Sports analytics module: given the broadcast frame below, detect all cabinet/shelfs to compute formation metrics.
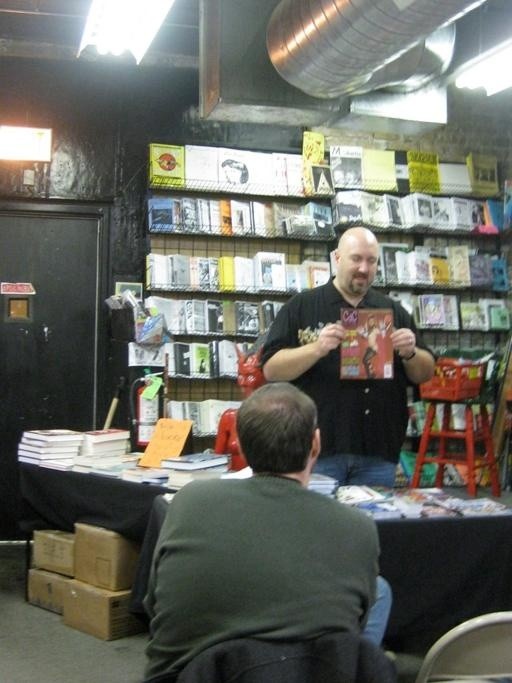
[141,142,512,477]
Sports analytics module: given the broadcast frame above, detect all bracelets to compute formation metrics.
[406,351,416,364]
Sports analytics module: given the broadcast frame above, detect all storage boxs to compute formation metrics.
[61,578,138,640]
[32,528,75,578]
[75,522,138,590]
[26,568,74,615]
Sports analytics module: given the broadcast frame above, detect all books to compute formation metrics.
[148,196,511,237]
[424,334,502,352]
[503,179,511,237]
[402,387,492,433]
[379,239,511,289]
[15,427,254,480]
[150,143,336,196]
[388,292,512,331]
[310,472,511,517]
[167,341,250,380]
[386,148,500,195]
[147,298,285,334]
[165,397,241,433]
[339,307,395,381]
[396,447,503,487]
[328,145,399,192]
[144,250,330,292]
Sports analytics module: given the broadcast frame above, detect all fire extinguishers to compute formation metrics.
[130,368,165,448]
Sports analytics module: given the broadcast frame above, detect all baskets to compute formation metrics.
[419,357,483,402]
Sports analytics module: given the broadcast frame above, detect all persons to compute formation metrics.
[356,315,385,378]
[256,228,434,486]
[143,383,393,682]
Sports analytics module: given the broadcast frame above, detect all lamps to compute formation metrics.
[455,40,512,96]
[75,0,175,65]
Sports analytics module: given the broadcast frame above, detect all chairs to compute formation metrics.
[414,612,512,683]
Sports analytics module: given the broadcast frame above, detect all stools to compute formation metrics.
[409,399,500,498]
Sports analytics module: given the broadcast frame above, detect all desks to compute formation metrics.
[8,458,512,603]
